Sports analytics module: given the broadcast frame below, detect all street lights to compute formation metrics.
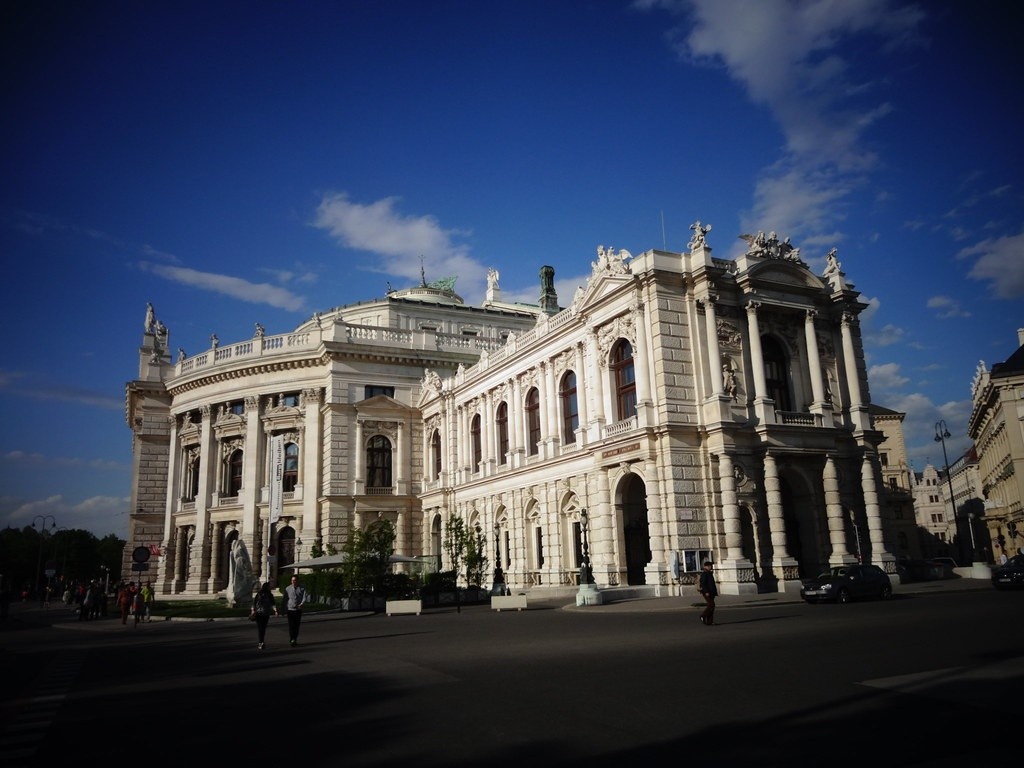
[579,508,596,584]
[32,515,56,592]
[934,420,965,555]
[493,521,507,585]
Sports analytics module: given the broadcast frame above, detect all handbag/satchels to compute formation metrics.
[247,613,256,623]
[115,600,119,608]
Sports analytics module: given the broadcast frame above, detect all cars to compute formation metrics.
[992,554,1024,590]
[800,564,893,605]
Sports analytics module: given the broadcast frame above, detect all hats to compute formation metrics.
[704,562,714,567]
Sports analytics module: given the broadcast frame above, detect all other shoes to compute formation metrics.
[708,621,719,625]
[147,620,152,623]
[122,622,126,624]
[699,615,707,625]
[288,640,297,647]
[141,621,145,623]
[258,642,264,649]
[136,618,139,624]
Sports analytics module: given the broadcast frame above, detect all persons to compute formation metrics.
[697,561,719,625]
[63,580,109,622]
[1000,550,1008,565]
[140,581,155,623]
[282,574,306,647]
[115,582,144,625]
[251,582,278,652]
[41,584,56,608]
